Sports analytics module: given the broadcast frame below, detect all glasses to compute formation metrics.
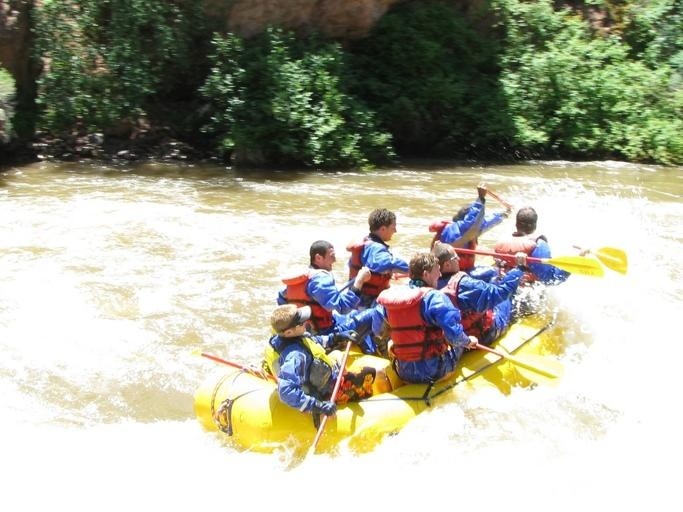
[450,252,458,260]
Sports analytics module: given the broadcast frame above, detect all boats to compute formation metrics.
[193,298,565,463]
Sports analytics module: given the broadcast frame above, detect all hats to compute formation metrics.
[277,305,311,333]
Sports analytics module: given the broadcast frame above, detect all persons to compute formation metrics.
[269,182,573,416]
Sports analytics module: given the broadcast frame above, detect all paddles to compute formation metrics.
[573,245,628,276]
[454,247,603,280]
[477,342,564,386]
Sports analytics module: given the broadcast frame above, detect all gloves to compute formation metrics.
[313,400,337,416]
[333,329,359,342]
